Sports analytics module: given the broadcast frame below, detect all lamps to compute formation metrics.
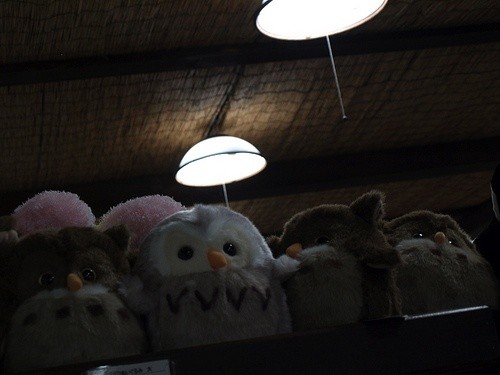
[174,136,269,187]
[254,0,390,42]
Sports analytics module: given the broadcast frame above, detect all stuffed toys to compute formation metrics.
[265,192,408,334]
[98,195,294,350]
[2,190,147,374]
[378,206,498,325]
